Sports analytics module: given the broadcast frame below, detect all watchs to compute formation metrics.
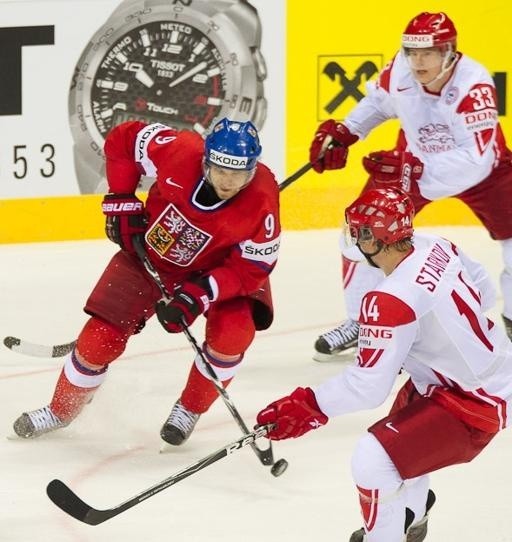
[69,0,268,195]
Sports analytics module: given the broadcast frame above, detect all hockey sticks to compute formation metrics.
[47,423,278,526]
[134,233,273,467]
[4,140,341,358]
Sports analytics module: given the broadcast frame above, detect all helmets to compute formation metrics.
[401,12,457,70]
[202,117,261,187]
[342,187,415,249]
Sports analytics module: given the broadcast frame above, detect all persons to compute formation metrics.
[309,11,512,363]
[6,117,281,454]
[256,185,512,542]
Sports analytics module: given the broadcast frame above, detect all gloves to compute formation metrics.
[253,388,328,441]
[155,277,212,333]
[101,195,150,259]
[309,120,350,173]
[362,152,424,186]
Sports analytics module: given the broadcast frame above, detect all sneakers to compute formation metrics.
[14,404,68,438]
[162,399,202,446]
[315,319,361,356]
[502,314,512,343]
[350,490,436,541]
